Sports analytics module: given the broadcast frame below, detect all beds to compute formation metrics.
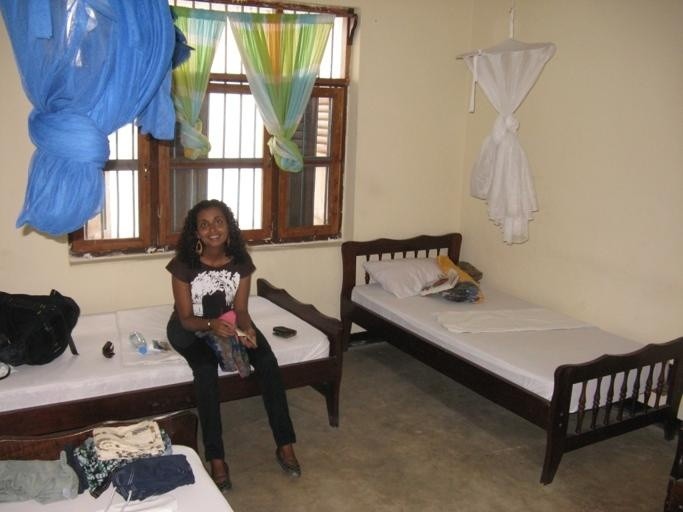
[0,279,344,426]
[0,411,236,512]
[341,233,683,485]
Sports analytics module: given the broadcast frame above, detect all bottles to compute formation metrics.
[128,330,148,355]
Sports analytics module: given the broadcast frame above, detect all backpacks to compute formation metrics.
[0,288,81,367]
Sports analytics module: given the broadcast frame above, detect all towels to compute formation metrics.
[115,303,183,367]
[431,307,598,335]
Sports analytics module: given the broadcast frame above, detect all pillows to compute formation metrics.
[364,258,447,297]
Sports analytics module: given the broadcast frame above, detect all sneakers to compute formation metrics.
[211,459,233,492]
[275,443,301,478]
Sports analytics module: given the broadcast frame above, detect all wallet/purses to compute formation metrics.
[273,325,295,339]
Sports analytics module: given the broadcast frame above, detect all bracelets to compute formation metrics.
[206,319,211,330]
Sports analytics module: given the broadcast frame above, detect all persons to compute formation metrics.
[163,199,303,492]
